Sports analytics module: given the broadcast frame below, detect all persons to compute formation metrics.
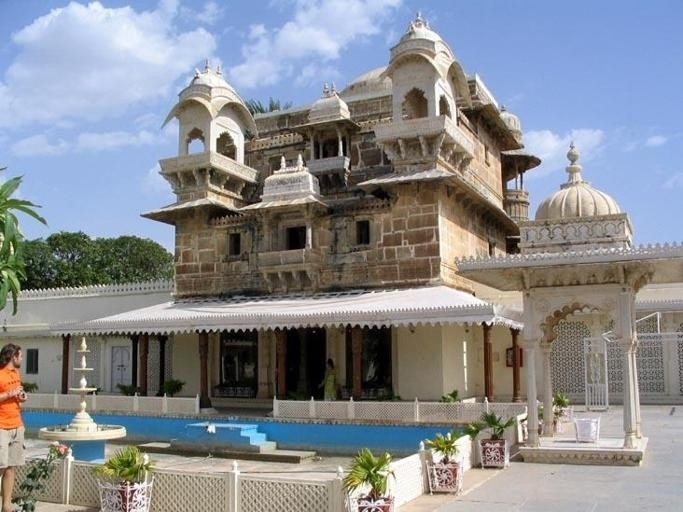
[319,358,338,400]
[0,343,27,512]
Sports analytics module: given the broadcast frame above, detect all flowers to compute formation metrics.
[9,443,71,512]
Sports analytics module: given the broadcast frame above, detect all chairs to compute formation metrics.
[570,414,600,444]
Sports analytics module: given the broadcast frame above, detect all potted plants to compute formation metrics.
[343,442,402,512]
[464,411,517,471]
[534,396,568,438]
[92,440,156,512]
[422,425,467,498]
